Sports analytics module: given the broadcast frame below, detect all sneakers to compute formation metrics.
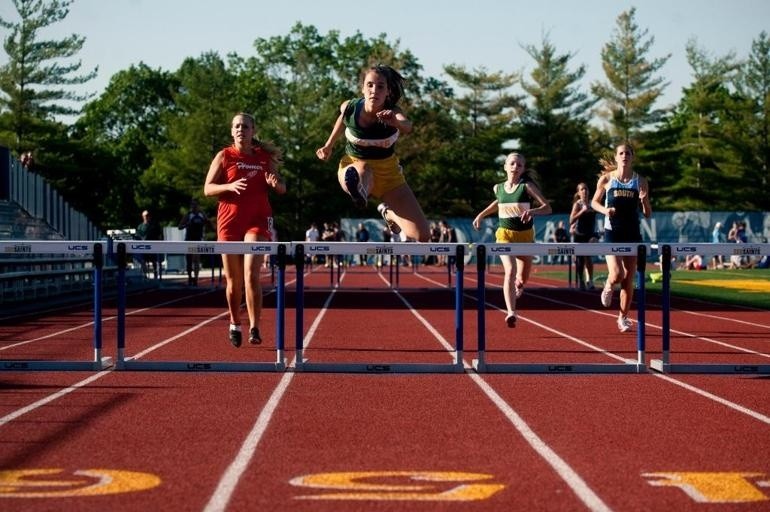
[584,279,634,332]
[378,202,403,235]
[507,282,524,328]
[344,166,370,210]
[228,322,260,349]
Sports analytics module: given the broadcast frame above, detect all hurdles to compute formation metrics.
[112,240,292,371]
[651,242,769,373]
[0,237,114,372]
[473,241,653,373]
[155,252,574,294]
[289,242,470,373]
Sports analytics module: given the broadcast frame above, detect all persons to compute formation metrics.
[179,199,223,285]
[553,221,569,264]
[316,65,431,242]
[137,210,161,281]
[473,152,553,327]
[591,144,650,332]
[305,222,346,267]
[264,254,270,268]
[383,219,456,275]
[570,183,595,290]
[660,222,769,272]
[204,114,286,349]
[356,223,370,266]
